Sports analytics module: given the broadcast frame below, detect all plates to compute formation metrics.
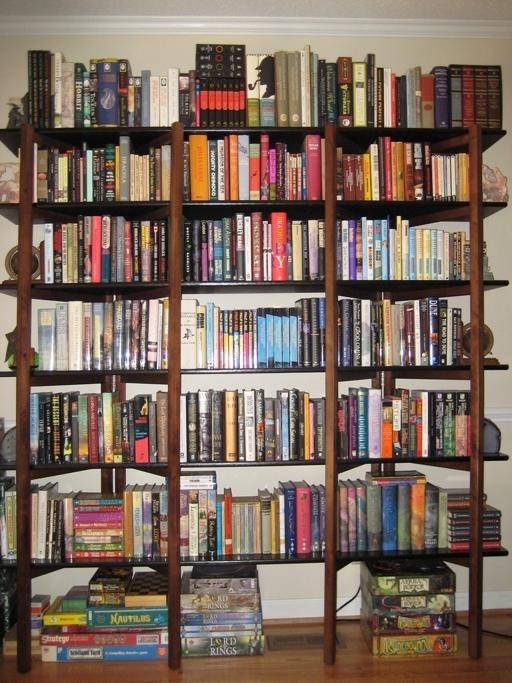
[4,245,41,280]
[463,322,495,358]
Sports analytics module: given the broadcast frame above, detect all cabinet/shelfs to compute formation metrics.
[0,125,509,673]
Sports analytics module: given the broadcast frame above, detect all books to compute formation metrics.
[180,565,265,657]
[3,566,168,662]
[0,42,503,129]
[335,214,502,553]
[180,212,325,556]
[359,559,457,657]
[1,135,471,203]
[31,216,167,558]
[1,476,17,560]
